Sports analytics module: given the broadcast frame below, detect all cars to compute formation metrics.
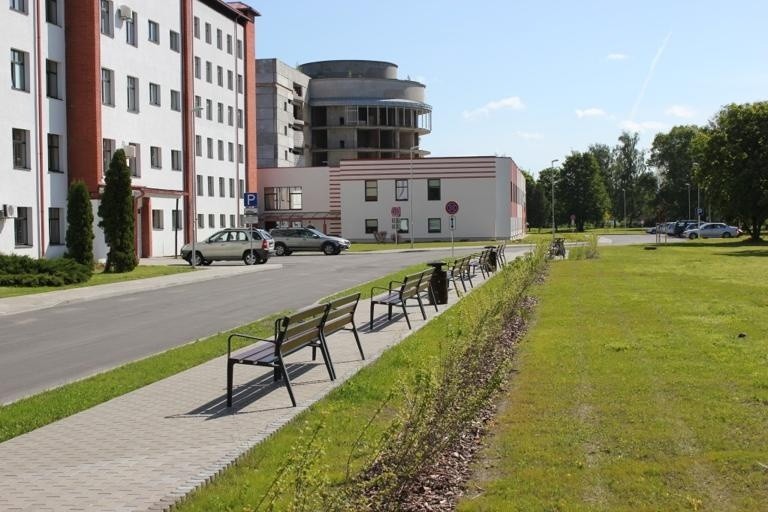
[646,220,743,239]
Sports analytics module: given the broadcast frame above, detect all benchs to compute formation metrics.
[368,244,510,334]
[225,292,365,410]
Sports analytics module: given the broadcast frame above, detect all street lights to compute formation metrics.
[622,189,626,228]
[551,160,558,238]
[685,183,691,219]
[410,145,431,248]
[180,227,351,266]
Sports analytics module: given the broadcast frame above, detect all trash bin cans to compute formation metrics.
[552,238,565,259]
[427,262,448,305]
[483,246,497,272]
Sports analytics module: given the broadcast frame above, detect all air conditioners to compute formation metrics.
[1,203,19,219]
[119,5,133,21]
[124,145,136,159]
